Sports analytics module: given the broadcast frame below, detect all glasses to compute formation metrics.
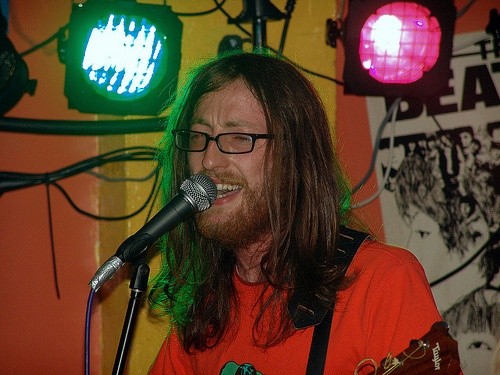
[169,128,287,153]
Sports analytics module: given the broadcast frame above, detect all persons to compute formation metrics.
[147,52,465,375]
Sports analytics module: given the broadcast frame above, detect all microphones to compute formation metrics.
[94,174,218,291]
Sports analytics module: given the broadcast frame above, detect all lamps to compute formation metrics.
[338,0,456,102]
[65,1,183,115]
